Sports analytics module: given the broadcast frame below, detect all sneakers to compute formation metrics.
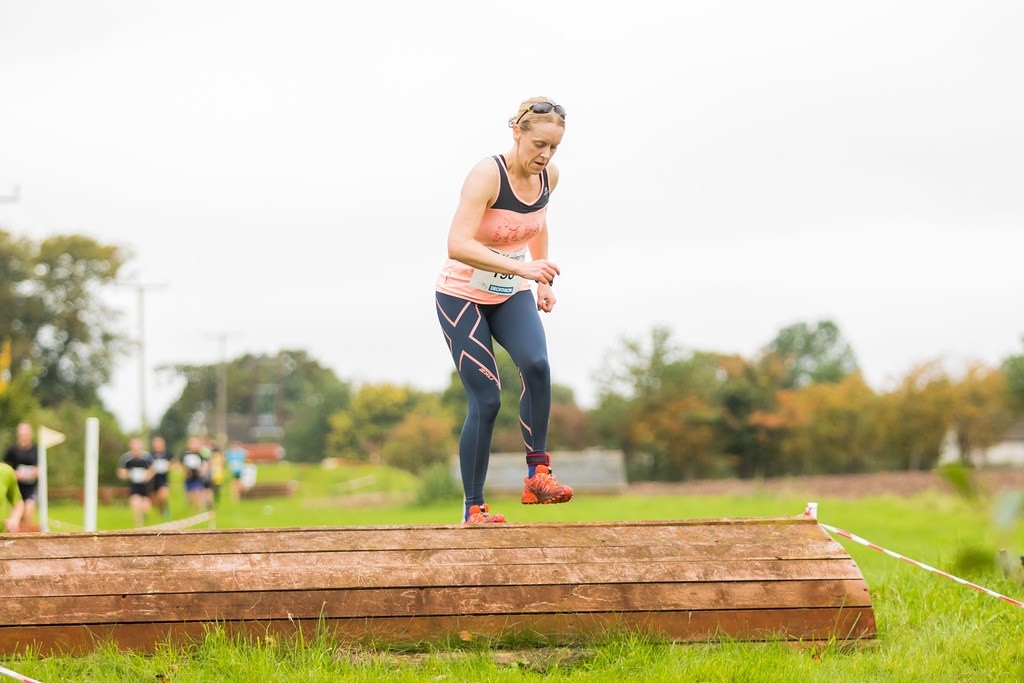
[463,504,506,525]
[521,465,573,504]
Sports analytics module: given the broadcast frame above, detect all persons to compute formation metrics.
[433,95,572,526]
[0,422,254,532]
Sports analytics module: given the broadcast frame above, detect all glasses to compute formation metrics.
[516,102,567,124]
